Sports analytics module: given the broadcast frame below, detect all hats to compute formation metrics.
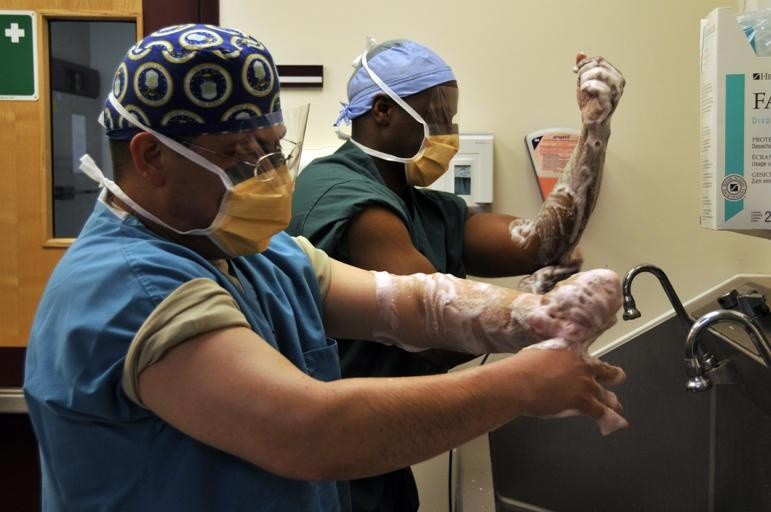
[328,34,458,127]
[96,20,284,140]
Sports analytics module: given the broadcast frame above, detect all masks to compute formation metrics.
[154,133,295,259]
[370,90,459,188]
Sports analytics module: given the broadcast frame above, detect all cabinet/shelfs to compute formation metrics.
[2,2,147,349]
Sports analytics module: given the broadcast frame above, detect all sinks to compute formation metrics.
[487,309,770,512]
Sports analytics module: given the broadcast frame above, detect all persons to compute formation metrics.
[21,21,625,512]
[281,40,625,511]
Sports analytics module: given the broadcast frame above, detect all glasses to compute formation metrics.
[179,140,301,182]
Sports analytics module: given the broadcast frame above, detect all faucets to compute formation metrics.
[686,309,771,394]
[623,263,716,371]
